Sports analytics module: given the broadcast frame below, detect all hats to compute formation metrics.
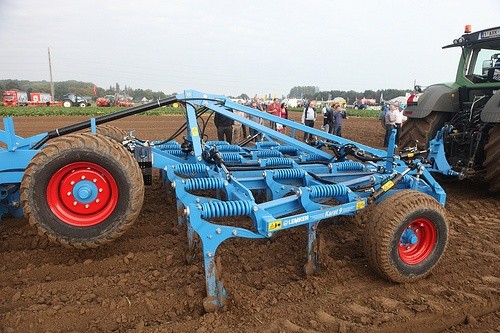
[399,104,405,109]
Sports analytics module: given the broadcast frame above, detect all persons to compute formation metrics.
[238,96,289,145]
[214,102,235,144]
[321,103,404,148]
[301,100,318,142]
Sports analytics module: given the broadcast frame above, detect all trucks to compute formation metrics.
[29,92,51,102]
[96,92,134,107]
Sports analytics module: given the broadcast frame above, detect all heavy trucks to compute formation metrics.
[1,89,28,108]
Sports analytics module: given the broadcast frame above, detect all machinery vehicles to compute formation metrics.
[62,93,91,108]
[397,23,500,196]
